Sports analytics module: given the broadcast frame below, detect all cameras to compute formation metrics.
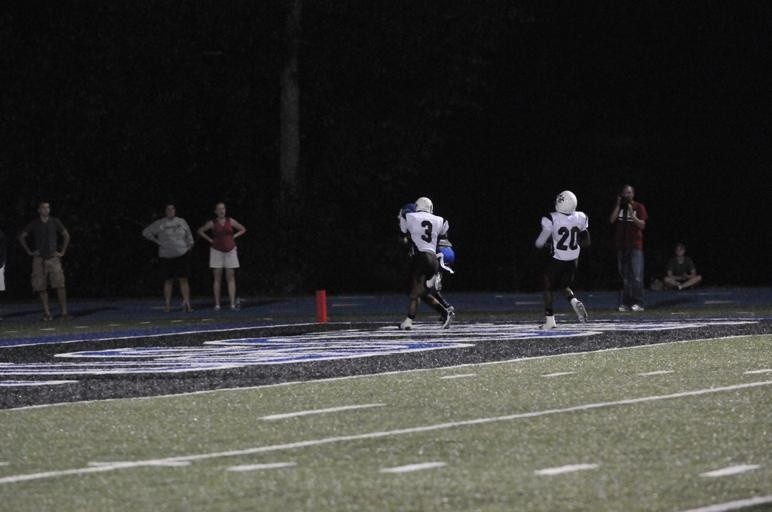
[621,196,629,208]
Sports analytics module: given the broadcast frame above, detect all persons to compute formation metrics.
[0,230,9,321]
[17,200,77,322]
[534,190,592,329]
[661,242,703,291]
[400,201,458,322]
[195,200,248,312]
[141,201,197,314]
[607,184,650,312]
[396,196,454,332]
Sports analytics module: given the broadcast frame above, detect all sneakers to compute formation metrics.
[537,324,557,331]
[398,322,413,331]
[617,304,630,314]
[441,305,455,329]
[59,313,71,323]
[212,307,223,314]
[575,301,589,324]
[230,305,241,312]
[630,304,644,313]
[182,302,195,314]
[162,302,172,315]
[43,313,53,323]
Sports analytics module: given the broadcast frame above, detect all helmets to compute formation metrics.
[554,190,577,215]
[414,196,434,214]
